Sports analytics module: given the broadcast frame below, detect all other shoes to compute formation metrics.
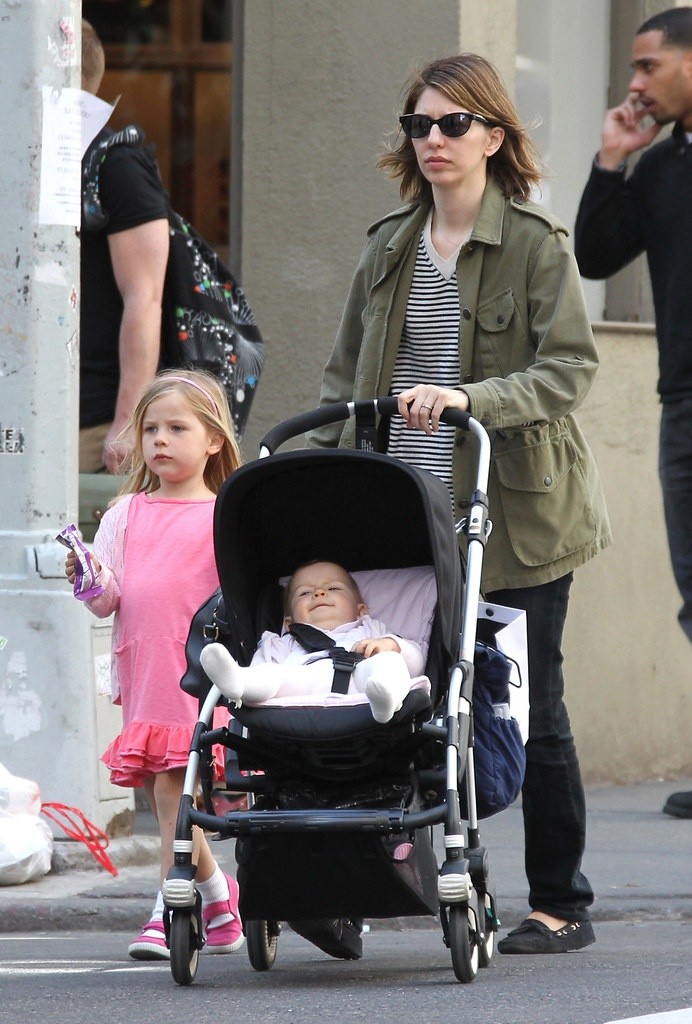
[498,919,597,954]
[288,911,367,960]
[663,790,692,818]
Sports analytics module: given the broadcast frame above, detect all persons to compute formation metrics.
[64,368,245,958]
[197,558,423,724]
[573,5,692,821]
[78,20,177,476]
[287,53,617,960]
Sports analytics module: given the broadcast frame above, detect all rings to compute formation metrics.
[421,404,432,411]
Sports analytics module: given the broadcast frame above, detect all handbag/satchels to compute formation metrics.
[468,642,526,818]
[82,124,264,444]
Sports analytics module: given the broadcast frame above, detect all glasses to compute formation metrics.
[399,112,493,137]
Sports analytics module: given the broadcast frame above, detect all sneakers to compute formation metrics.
[201,873,242,953]
[127,920,171,960]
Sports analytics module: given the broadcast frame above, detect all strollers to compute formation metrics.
[161,397,498,989]
